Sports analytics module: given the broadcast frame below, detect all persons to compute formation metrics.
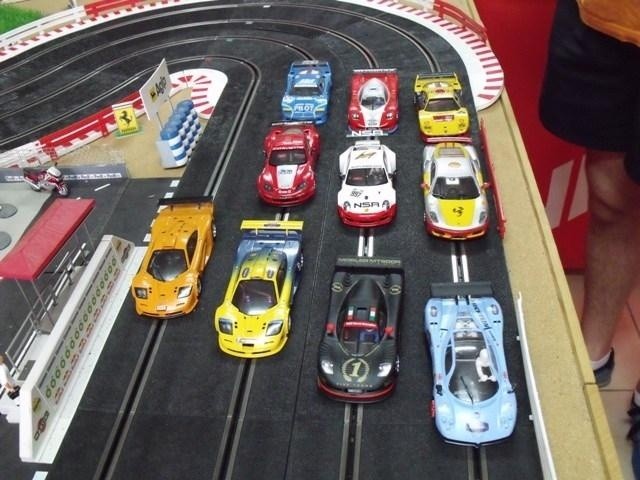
[0,354,20,400]
[538,0,640,480]
